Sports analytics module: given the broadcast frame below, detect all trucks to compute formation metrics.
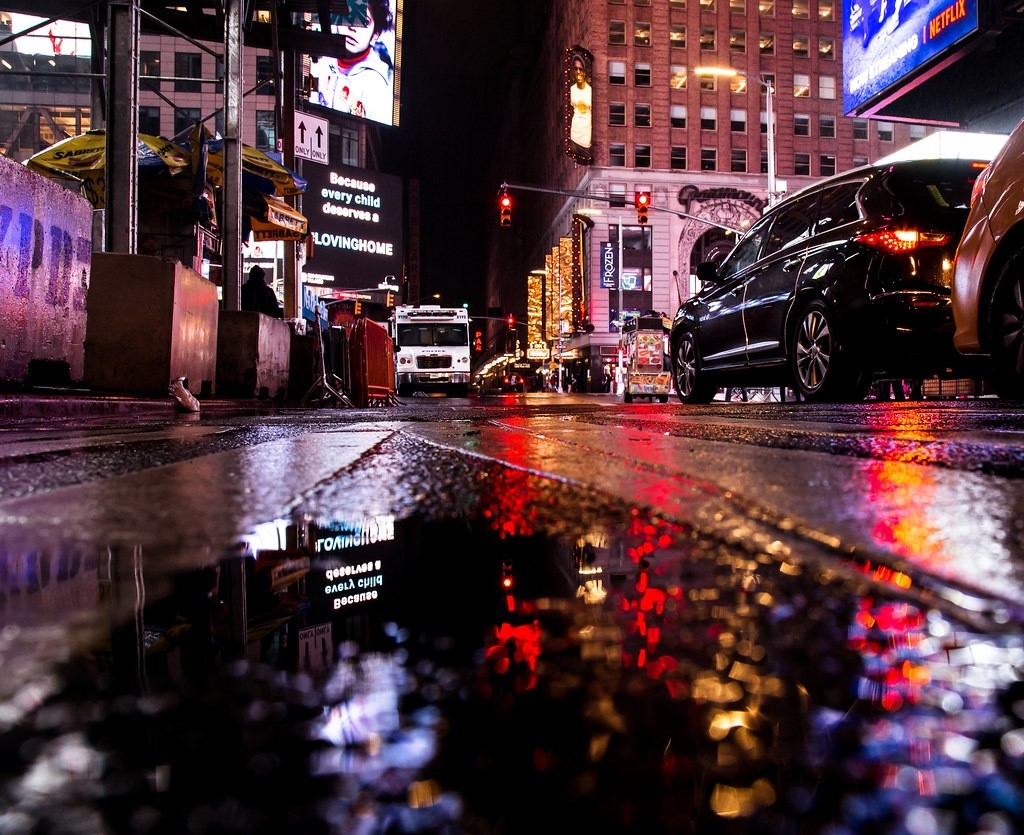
[394,305,472,398]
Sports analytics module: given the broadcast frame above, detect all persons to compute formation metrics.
[570,54,593,156]
[241,265,279,317]
[569,374,576,392]
[604,363,612,380]
[310,0,393,124]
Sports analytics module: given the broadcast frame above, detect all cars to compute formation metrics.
[503,375,524,392]
[949,115,1024,402]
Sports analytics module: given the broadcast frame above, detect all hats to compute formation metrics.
[249,265,265,279]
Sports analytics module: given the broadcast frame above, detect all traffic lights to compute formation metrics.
[463,303,468,308]
[500,196,512,228]
[508,318,513,329]
[637,194,647,223]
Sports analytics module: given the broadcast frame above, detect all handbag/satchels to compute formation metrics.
[22,357,71,394]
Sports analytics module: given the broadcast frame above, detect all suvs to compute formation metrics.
[667,157,991,403]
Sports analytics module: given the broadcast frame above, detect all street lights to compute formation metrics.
[531,270,563,394]
[697,67,774,211]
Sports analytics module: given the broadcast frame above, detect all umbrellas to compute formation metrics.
[22,122,313,243]
